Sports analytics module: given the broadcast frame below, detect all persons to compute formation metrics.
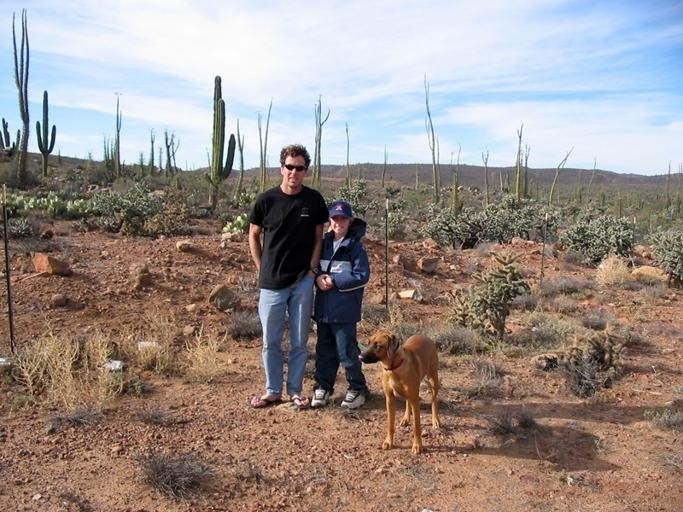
[246,144,329,408]
[309,201,369,411]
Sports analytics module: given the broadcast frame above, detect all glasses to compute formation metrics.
[284,163,307,172]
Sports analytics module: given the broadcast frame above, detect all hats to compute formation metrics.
[330,201,351,218]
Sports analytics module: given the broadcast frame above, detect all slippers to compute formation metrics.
[250,397,311,409]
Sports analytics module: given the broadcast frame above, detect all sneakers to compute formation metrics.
[310,384,370,410]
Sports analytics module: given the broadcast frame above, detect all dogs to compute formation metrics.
[358,330,441,453]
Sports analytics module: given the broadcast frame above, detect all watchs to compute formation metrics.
[310,265,319,274]
